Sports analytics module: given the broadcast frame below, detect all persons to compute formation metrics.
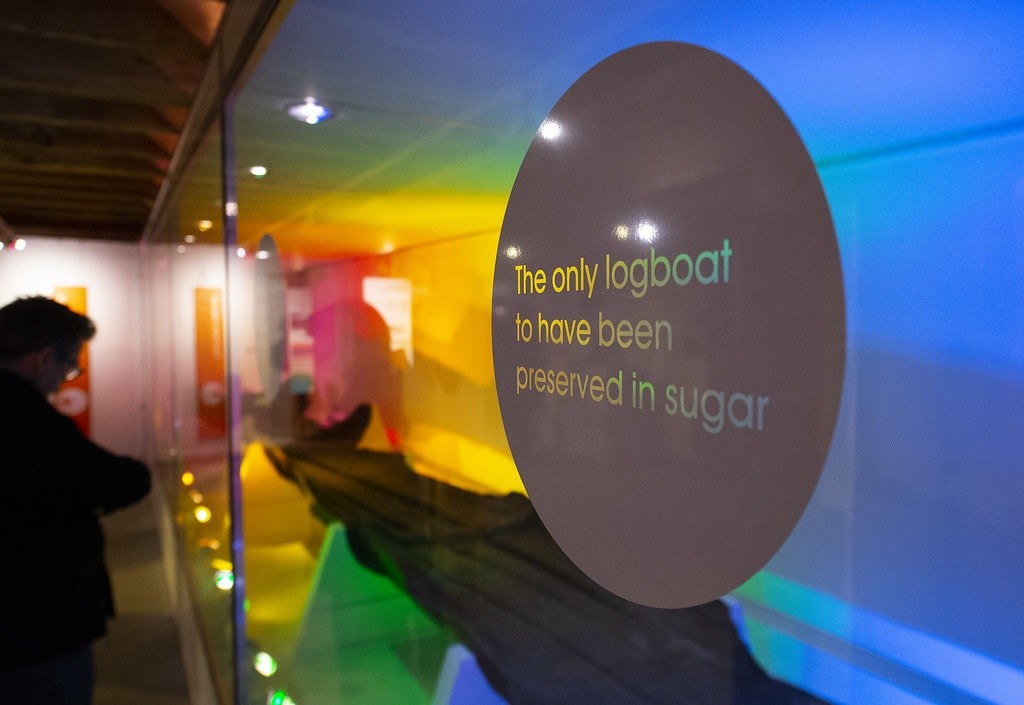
[260,294,433,596]
[0,294,153,705]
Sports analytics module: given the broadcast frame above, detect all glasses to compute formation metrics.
[55,350,81,382]
